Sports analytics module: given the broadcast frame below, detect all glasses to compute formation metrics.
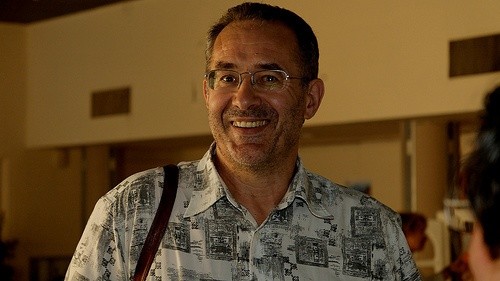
[206,68,309,95]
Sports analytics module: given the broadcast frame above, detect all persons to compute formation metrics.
[398,212,469,281]
[460,84,500,281]
[64,1,422,281]
